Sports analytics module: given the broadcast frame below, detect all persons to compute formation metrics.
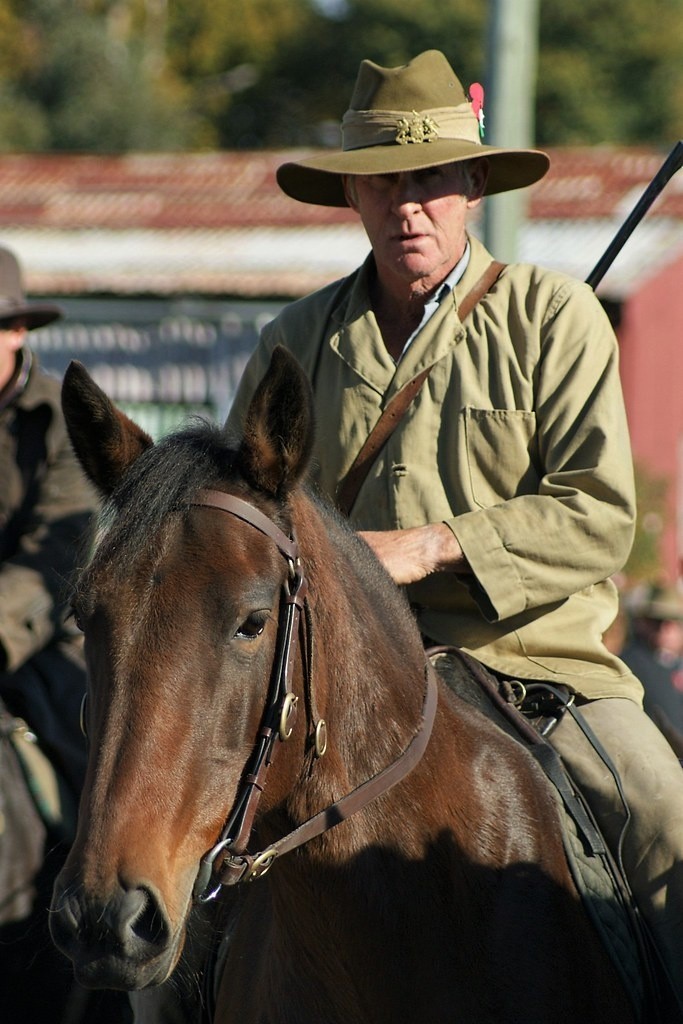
[0,248,98,795]
[618,584,683,767]
[221,49,683,1004]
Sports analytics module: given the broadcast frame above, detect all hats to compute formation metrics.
[0,249,63,330]
[276,48,551,208]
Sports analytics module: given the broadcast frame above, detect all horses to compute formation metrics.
[45,345,681,1023]
